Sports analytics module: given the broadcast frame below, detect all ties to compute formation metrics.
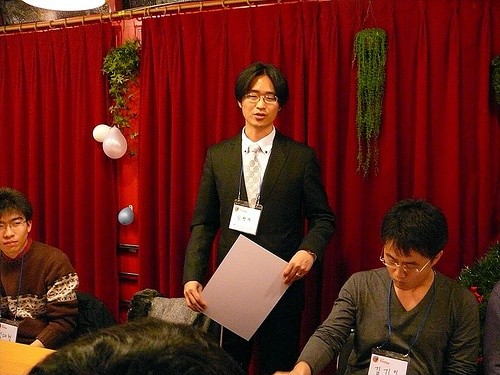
[245,145,261,207]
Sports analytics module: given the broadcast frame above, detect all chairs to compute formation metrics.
[76,292,112,334]
[126,288,217,332]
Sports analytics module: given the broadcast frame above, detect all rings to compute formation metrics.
[296,273,300,276]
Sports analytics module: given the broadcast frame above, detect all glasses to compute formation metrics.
[244,94,278,105]
[0,220,27,233]
[380,248,434,277]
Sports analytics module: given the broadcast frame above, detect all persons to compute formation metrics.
[483,279,500,375]
[182,61,336,375]
[28,317,247,375]
[0,188,81,349]
[276,198,483,375]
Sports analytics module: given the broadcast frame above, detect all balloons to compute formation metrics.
[93,124,111,142]
[103,127,127,159]
[118,207,133,224]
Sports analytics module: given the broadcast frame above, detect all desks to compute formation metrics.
[0,339,57,375]
